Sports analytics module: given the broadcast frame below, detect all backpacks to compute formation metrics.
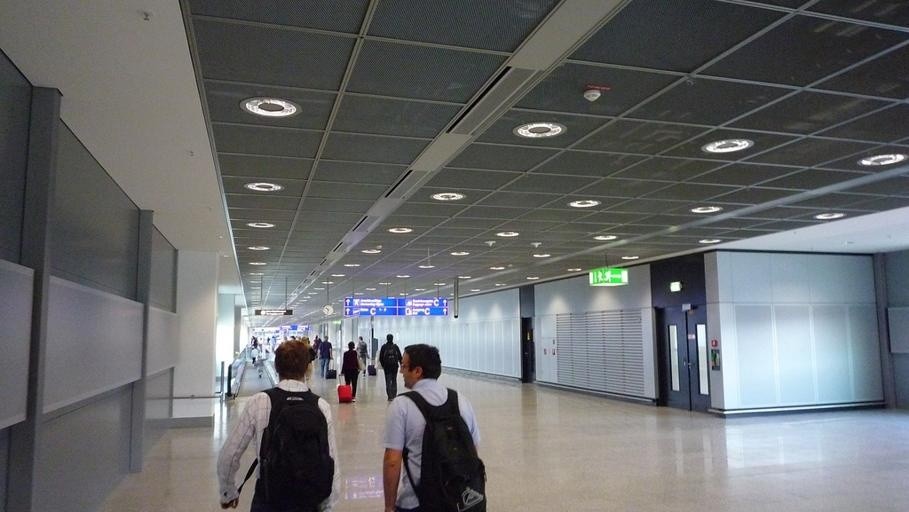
[261,388,335,511]
[397,387,486,512]
[383,344,398,367]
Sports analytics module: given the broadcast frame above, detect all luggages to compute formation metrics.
[368,365,376,375]
[326,369,336,380]
[337,375,352,402]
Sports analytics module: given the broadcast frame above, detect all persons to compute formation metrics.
[250,334,405,405]
[216,339,342,512]
[382,343,486,511]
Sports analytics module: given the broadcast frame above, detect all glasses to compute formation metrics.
[310,348,317,364]
[401,364,406,370]
